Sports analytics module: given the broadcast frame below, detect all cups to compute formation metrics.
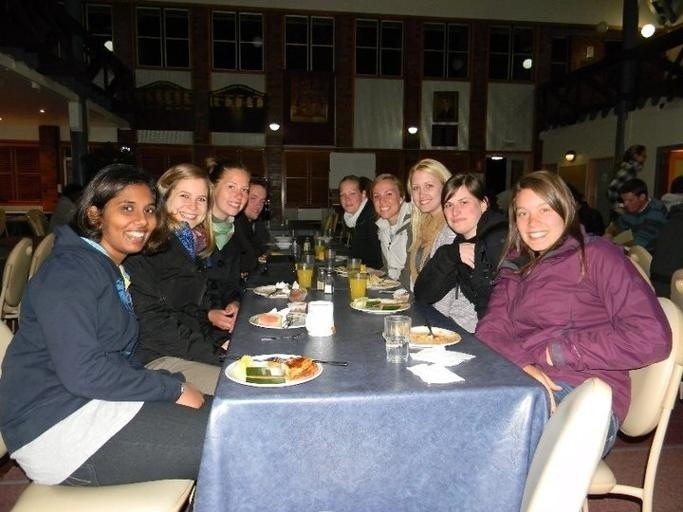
[345,257,361,272]
[313,240,336,269]
[304,299,335,338]
[347,272,367,298]
[295,262,313,288]
[383,315,411,362]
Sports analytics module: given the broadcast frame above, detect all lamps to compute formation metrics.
[431,90,458,122]
[562,149,575,163]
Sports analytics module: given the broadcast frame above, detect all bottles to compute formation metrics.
[316,269,334,294]
[291,235,310,258]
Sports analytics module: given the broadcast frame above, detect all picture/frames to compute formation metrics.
[287,72,331,124]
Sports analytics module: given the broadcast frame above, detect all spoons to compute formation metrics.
[425,319,435,337]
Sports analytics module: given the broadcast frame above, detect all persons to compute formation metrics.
[336,175,383,270]
[0,164,214,488]
[369,173,429,290]
[414,173,516,320]
[205,155,223,182]
[406,158,478,335]
[562,145,683,299]
[474,170,672,459]
[122,163,231,396]
[46,184,82,235]
[206,154,251,335]
[235,180,269,273]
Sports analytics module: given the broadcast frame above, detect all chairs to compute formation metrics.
[514,245,682,512]
[0,205,192,512]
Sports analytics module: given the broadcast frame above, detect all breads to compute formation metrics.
[288,288,306,301]
[257,314,278,326]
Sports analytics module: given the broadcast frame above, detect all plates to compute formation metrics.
[223,353,322,387]
[335,254,347,262]
[273,234,295,250]
[349,298,411,315]
[407,325,464,347]
[248,311,307,330]
[339,266,386,278]
[366,278,400,290]
[252,285,307,299]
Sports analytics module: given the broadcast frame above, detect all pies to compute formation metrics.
[283,358,318,380]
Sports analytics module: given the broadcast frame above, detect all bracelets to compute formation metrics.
[175,384,184,401]
